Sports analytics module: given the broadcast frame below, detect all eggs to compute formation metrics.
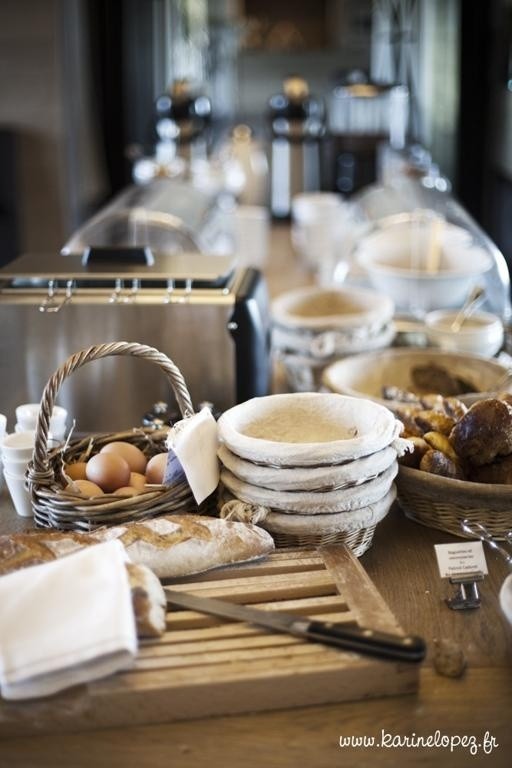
[61,441,170,498]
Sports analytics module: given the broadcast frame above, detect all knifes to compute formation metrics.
[156,587,424,664]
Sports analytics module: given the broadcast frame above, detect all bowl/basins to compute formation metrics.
[265,191,502,394]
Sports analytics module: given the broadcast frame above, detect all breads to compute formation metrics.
[0,510,275,635]
[384,382,511,483]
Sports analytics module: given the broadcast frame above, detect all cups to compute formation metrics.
[1,403,70,519]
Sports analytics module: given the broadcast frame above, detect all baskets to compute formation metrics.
[394,452,512,545]
[208,390,403,557]
[21,340,202,533]
